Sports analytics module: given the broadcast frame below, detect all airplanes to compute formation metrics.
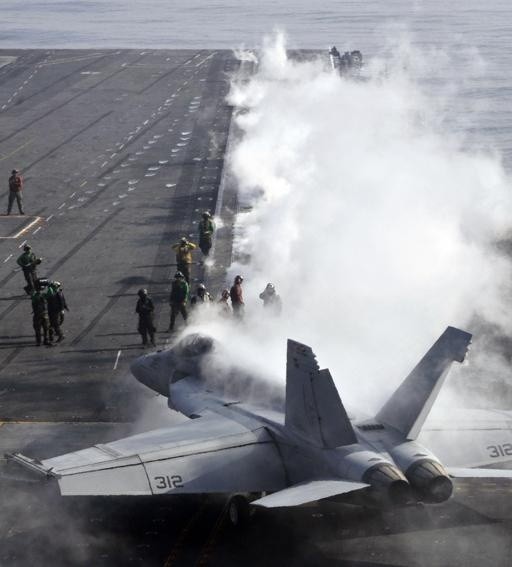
[3,326,512,532]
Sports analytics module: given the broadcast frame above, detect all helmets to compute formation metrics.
[234,275,244,284]
[200,212,211,218]
[10,169,19,174]
[221,290,230,298]
[266,283,275,290]
[23,244,32,250]
[137,288,148,296]
[174,271,185,277]
[179,237,187,243]
[197,284,206,290]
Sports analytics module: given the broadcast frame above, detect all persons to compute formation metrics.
[16,244,41,295]
[171,237,197,288]
[136,288,157,346]
[197,211,214,256]
[32,277,70,347]
[6,170,25,215]
[166,271,282,333]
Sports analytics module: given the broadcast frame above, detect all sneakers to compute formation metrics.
[166,328,176,334]
[141,338,157,348]
[24,286,33,295]
[7,208,25,216]
[37,335,65,346]
